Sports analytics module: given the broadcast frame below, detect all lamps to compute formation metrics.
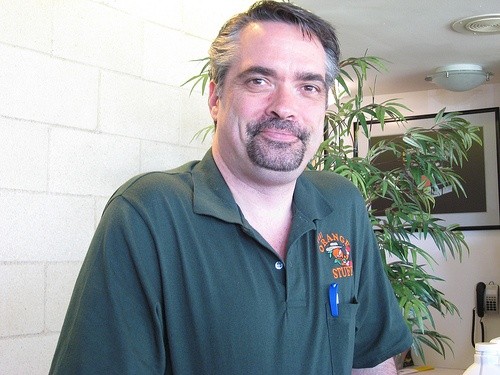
[426,65,495,92]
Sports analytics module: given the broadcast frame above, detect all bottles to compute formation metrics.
[474,343,500,375]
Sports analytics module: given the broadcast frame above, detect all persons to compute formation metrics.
[48,0,414,375]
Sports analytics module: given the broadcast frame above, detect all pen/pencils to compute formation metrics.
[329,283,340,317]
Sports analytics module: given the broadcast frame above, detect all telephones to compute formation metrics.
[476,281,499,318]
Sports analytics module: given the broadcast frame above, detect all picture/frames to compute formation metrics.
[354,106,500,234]
